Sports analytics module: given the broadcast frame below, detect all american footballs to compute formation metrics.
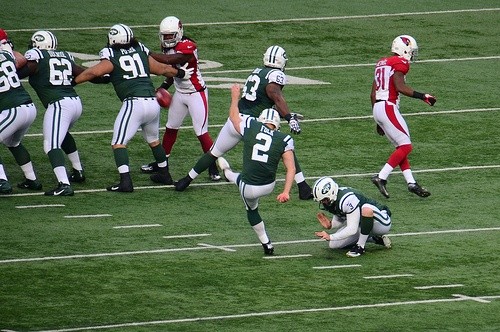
[156,88,172,108]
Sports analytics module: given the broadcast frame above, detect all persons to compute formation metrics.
[174,46,313,199]
[139,17,221,181]
[371,35,437,198]
[310,177,392,258]
[0,29,42,193]
[71,23,194,191]
[218,82,296,255]
[15,31,111,196]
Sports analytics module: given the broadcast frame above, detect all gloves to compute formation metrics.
[142,43,152,55]
[413,91,436,106]
[377,124,385,136]
[284,113,300,135]
[176,62,194,79]
[290,112,304,119]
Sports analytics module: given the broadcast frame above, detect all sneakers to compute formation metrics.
[107,184,133,192]
[346,243,366,257]
[17,178,42,190]
[379,235,391,248]
[371,174,390,198]
[140,157,169,174]
[408,182,434,196]
[175,179,186,191]
[265,244,274,255]
[0,179,13,194]
[44,181,75,196]
[151,172,172,184]
[218,157,231,182]
[68,167,85,182]
[210,174,221,181]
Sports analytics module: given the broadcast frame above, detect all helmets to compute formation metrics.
[31,30,58,50]
[263,45,288,71]
[0,28,8,43]
[392,35,418,63]
[159,15,183,47]
[313,176,339,203]
[107,23,134,47]
